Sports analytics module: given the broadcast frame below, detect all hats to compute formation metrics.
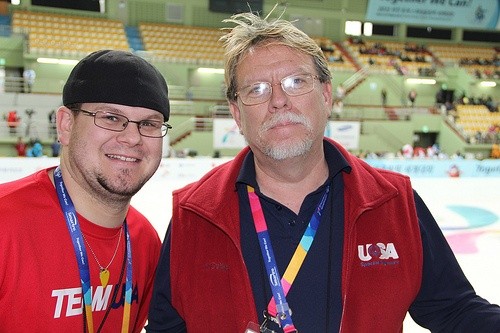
[62,50,170,122]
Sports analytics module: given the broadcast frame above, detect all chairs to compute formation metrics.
[311,36,500,73]
[459,104,500,139]
[11,9,134,60]
[141,19,229,60]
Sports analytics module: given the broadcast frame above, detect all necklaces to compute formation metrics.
[81,227,122,288]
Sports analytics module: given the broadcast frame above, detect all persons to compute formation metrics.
[319,33,500,161]
[22,65,37,92]
[0,50,173,333]
[6,106,61,157]
[145,12,500,333]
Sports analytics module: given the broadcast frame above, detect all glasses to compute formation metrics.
[72,109,172,137]
[233,73,319,107]
